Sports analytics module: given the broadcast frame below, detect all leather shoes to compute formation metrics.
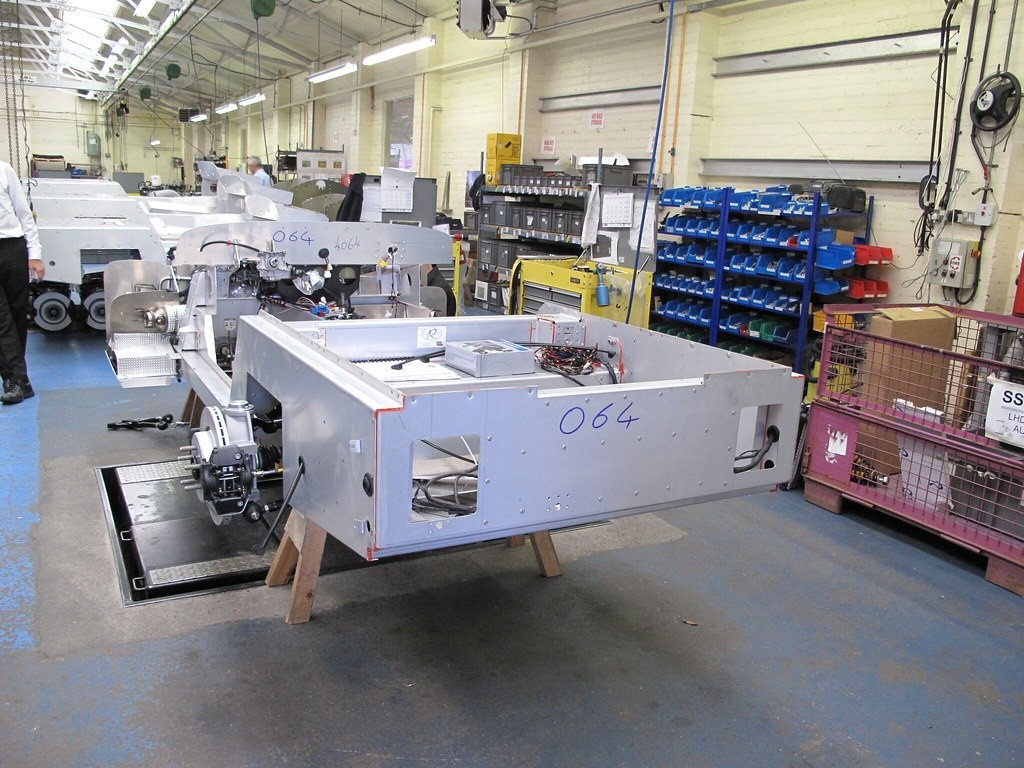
[0,379,34,403]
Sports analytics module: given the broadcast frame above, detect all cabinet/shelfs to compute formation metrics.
[276,143,345,184]
[476,165,635,316]
[713,186,874,373]
[647,200,747,345]
[799,302,1024,598]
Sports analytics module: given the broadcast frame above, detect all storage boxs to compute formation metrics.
[648,185,894,365]
[475,272,511,307]
[482,200,583,237]
[484,132,584,188]
[856,305,958,475]
[948,427,1024,541]
[479,237,551,269]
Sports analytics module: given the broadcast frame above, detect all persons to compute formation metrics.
[0,160,45,403]
[248,156,273,187]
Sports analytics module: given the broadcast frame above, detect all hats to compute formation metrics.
[248,156,260,165]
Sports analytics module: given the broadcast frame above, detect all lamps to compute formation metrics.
[86,37,129,98]
[133,0,157,17]
[214,103,239,114]
[362,34,437,65]
[190,114,208,123]
[307,63,358,83]
[238,94,267,106]
